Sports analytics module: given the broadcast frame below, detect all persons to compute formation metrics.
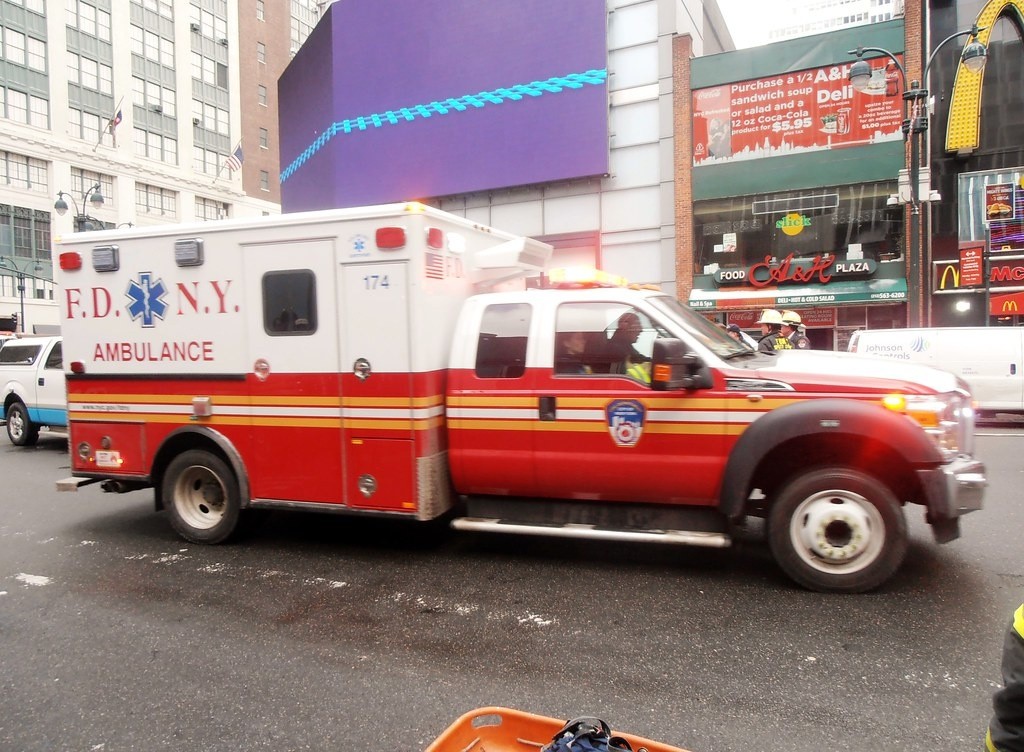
[756,310,795,351]
[607,312,652,374]
[781,311,811,350]
[726,324,754,351]
[555,332,603,374]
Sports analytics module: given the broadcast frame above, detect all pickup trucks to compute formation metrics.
[846,321,1024,424]
[0,334,64,448]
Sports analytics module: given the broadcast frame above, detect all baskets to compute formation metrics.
[421,704,698,752]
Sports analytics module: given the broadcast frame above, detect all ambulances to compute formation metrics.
[58,204,988,595]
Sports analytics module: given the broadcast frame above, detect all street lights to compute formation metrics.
[0,254,49,332]
[55,184,105,232]
[842,25,987,324]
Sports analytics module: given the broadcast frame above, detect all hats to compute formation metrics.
[725,323,741,334]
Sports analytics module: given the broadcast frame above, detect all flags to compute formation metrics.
[113,110,122,128]
[227,146,244,173]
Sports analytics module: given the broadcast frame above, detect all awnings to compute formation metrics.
[689,278,908,311]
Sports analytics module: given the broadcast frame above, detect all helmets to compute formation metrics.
[755,309,789,327]
[780,310,807,329]
